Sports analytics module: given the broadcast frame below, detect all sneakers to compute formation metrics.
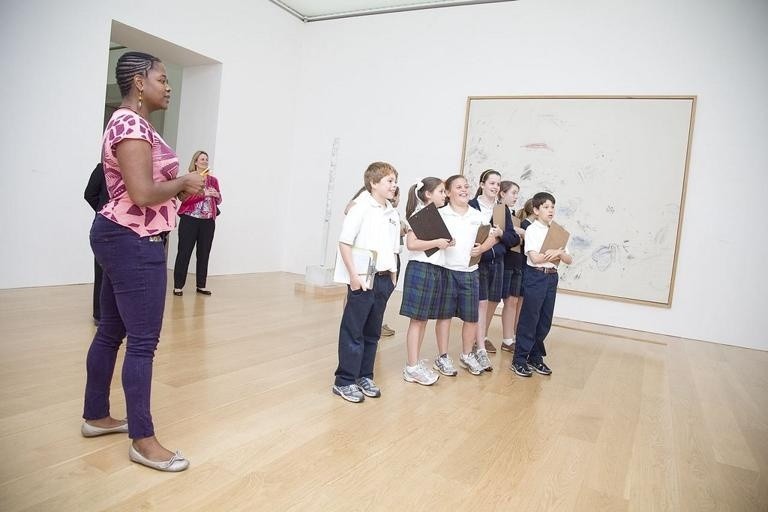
[172,287,184,297]
[484,336,497,354]
[526,359,553,375]
[509,360,532,377]
[355,375,382,398]
[500,340,516,354]
[330,381,366,404]
[126,442,192,474]
[381,324,396,337]
[401,358,440,387]
[474,347,494,372]
[194,288,213,296]
[432,351,459,377]
[458,350,485,375]
[79,415,131,439]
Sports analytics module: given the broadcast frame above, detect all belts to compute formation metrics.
[533,266,558,274]
[377,270,392,277]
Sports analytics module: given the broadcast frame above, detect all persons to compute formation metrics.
[81,51,206,472]
[173,151,222,296]
[84,162,110,327]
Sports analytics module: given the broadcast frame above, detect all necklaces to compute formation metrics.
[119,105,145,118]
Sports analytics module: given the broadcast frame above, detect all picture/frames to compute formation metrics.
[456,94,699,309]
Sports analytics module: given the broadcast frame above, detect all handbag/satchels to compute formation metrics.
[209,175,222,217]
